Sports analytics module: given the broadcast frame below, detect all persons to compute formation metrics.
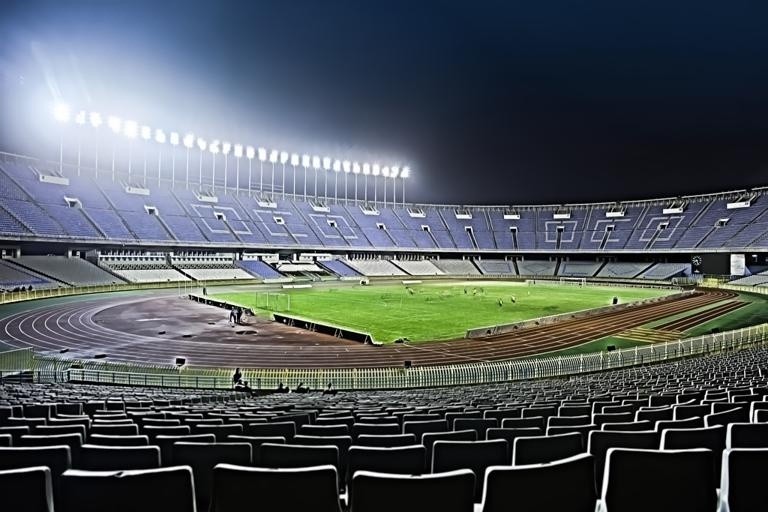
[511,294,516,305]
[463,285,467,295]
[229,307,242,324]
[472,288,477,297]
[498,297,504,308]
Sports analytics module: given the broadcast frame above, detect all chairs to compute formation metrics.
[1,340,767,511]
